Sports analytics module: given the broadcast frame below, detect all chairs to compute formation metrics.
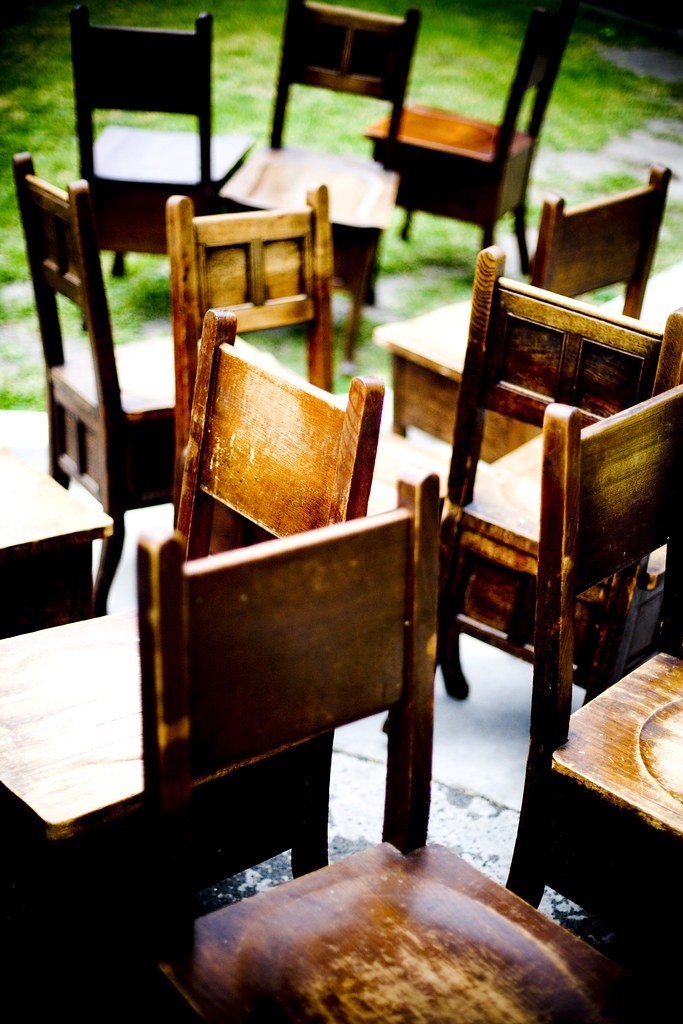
[0,0,683,1024]
